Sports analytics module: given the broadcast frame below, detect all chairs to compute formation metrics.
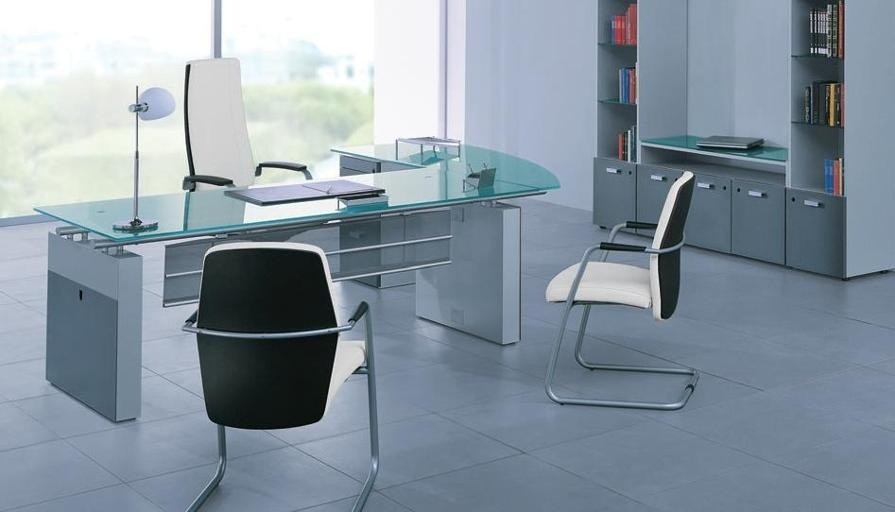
[182,58,312,193]
[544,171,700,410]
[180,241,380,511]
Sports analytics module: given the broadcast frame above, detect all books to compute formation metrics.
[803,1,844,197]
[608,2,637,164]
[224,177,387,207]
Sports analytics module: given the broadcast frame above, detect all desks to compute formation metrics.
[34,137,561,424]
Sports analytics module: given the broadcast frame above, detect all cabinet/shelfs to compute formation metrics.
[592,1,894,282]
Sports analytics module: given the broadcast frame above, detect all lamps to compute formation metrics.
[114,85,176,231]
